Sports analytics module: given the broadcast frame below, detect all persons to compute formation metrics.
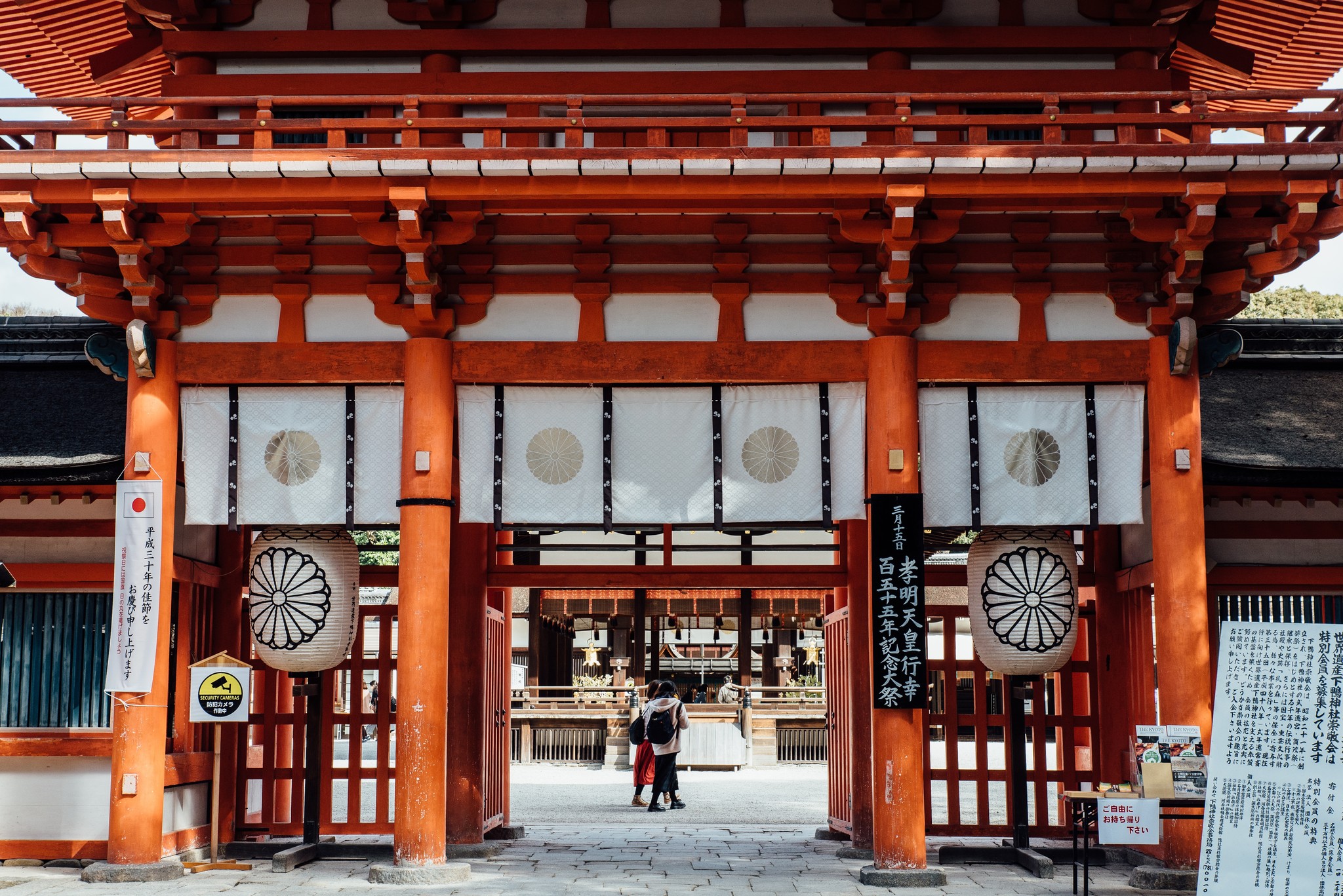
[362,679,371,742]
[694,686,702,703]
[368,680,378,740]
[697,683,709,703]
[631,678,681,808]
[717,675,739,704]
[647,681,690,813]
[683,684,696,703]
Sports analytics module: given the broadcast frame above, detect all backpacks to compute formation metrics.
[629,706,647,745]
[646,702,676,744]
[693,696,702,703]
[723,685,738,704]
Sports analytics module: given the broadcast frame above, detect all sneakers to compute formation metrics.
[632,795,651,807]
[662,792,680,805]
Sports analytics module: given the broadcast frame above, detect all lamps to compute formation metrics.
[581,613,602,666]
[803,617,822,665]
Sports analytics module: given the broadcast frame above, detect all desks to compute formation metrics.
[1057,794,1206,896]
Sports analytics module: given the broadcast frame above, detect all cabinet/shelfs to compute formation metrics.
[1129,735,1207,798]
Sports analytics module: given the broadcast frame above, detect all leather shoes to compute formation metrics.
[648,803,666,812]
[670,799,686,809]
